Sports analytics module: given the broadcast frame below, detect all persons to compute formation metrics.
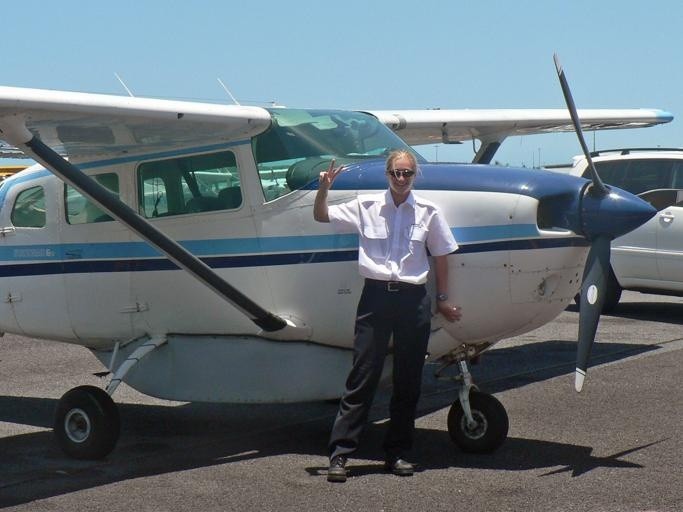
[312,150,463,482]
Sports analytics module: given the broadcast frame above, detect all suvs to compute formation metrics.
[564,142,683,312]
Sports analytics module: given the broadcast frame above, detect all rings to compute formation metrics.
[453,306,456,309]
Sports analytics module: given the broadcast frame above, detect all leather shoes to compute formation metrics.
[328,457,346,482]
[385,455,414,475]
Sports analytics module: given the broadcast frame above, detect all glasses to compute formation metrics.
[387,169,416,178]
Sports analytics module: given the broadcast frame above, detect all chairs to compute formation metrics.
[186,186,241,213]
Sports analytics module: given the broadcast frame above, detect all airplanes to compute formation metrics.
[0,52,678,465]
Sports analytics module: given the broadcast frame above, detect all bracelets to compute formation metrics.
[436,292,448,301]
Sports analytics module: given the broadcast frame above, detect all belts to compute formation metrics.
[365,278,418,292]
